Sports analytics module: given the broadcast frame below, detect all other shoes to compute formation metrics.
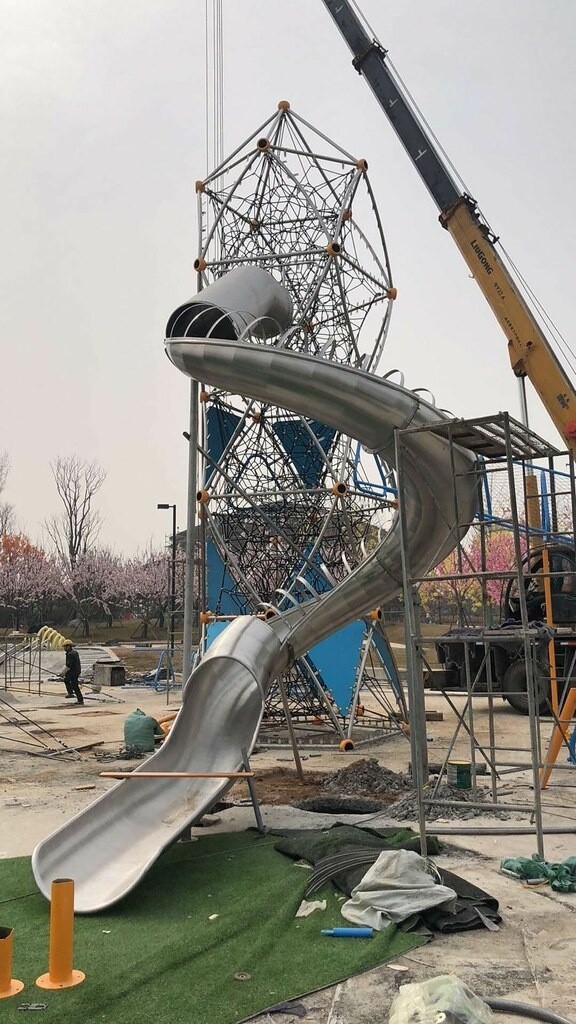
[74,699,84,704]
[65,692,74,698]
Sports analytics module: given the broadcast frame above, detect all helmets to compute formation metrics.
[63,640,73,647]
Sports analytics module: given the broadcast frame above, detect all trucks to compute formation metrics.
[422,539,576,717]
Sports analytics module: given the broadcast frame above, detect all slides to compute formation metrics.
[27,264,484,920]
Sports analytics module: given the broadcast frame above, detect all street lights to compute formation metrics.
[158,503,177,658]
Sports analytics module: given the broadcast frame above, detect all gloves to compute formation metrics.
[59,666,70,677]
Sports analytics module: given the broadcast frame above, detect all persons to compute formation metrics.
[57,639,84,705]
